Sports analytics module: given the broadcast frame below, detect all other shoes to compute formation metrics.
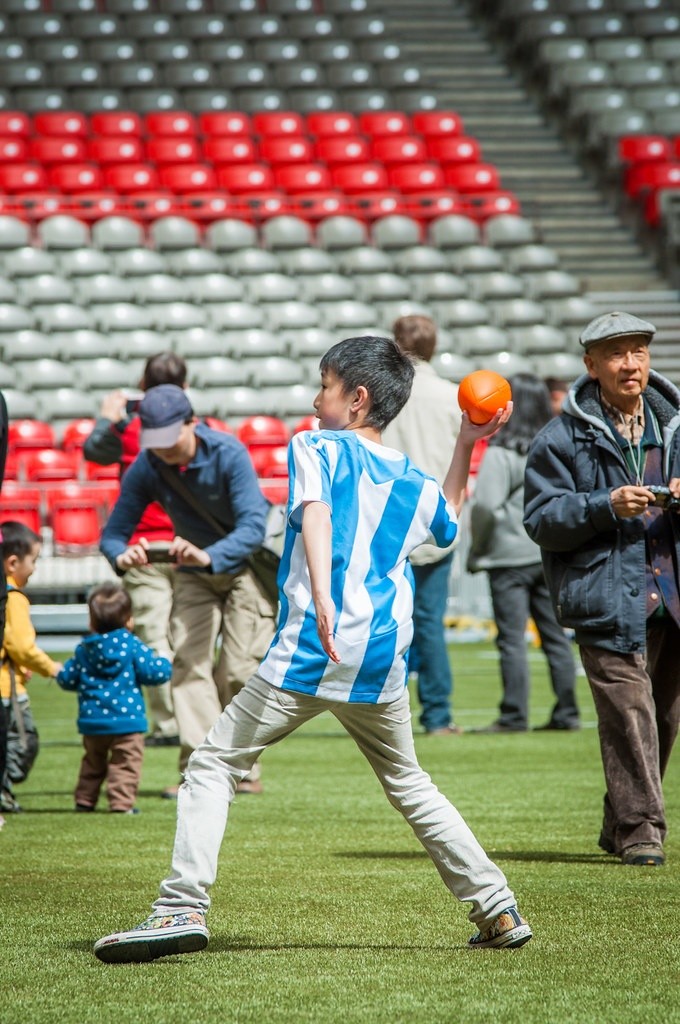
[472,722,527,733]
[533,723,577,731]
[162,780,184,799]
[429,726,461,736]
[75,802,94,812]
[113,808,140,814]
[236,779,262,793]
[598,829,616,854]
[622,843,666,865]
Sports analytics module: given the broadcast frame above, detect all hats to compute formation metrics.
[139,385,193,450]
[579,311,656,350]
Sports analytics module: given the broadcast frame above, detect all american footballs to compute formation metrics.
[459,370,513,426]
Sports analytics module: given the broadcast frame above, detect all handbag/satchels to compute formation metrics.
[250,504,287,602]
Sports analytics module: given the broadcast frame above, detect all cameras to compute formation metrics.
[122,393,144,417]
[646,485,680,512]
[144,542,177,563]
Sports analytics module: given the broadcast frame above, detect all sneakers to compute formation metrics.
[468,909,533,949]
[94,912,209,964]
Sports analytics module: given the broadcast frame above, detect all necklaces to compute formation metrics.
[617,411,643,486]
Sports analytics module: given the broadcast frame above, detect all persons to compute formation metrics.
[55,588,172,814]
[94,337,532,961]
[0,314,680,863]
[467,374,582,733]
